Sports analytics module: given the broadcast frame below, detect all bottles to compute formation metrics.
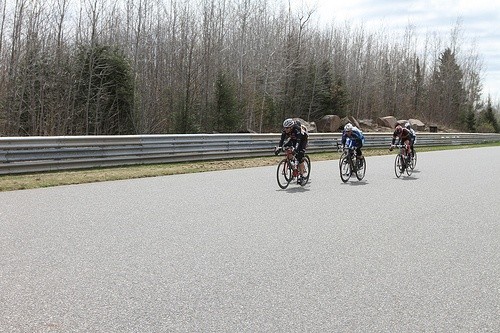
[351,156,356,165]
[290,160,295,166]
[295,160,298,169]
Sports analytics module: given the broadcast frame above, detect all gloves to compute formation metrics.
[275,150,280,155]
[292,149,299,156]
[389,145,394,151]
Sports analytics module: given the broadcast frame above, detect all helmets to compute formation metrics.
[405,123,411,127]
[283,118,295,127]
[396,125,402,132]
[344,123,353,131]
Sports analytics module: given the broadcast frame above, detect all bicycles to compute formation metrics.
[337,142,366,183]
[388,142,417,178]
[275,144,311,189]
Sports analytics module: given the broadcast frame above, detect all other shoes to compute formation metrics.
[407,154,412,162]
[281,168,288,175]
[300,162,305,174]
[359,156,364,166]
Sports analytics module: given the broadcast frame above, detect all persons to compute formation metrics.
[277,118,309,179]
[390,122,416,169]
[341,123,365,169]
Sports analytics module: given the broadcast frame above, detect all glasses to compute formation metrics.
[346,131,350,132]
[285,127,290,129]
